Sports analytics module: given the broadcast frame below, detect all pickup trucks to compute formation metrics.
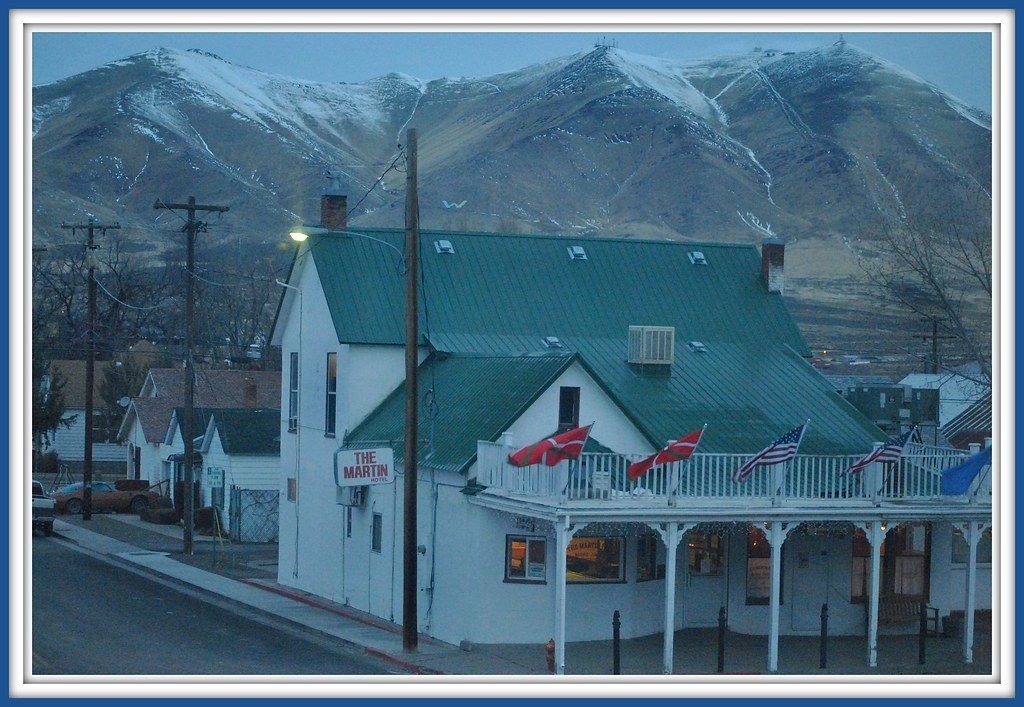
[32,479,57,535]
[49,481,162,514]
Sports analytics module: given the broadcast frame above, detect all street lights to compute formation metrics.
[291,224,418,652]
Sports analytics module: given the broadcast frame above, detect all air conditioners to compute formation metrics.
[335,484,361,505]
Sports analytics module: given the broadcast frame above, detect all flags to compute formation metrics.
[507,425,591,467]
[733,425,804,488]
[941,445,992,495]
[838,431,909,477]
[629,430,702,479]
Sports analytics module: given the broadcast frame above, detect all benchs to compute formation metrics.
[864,593,939,637]
